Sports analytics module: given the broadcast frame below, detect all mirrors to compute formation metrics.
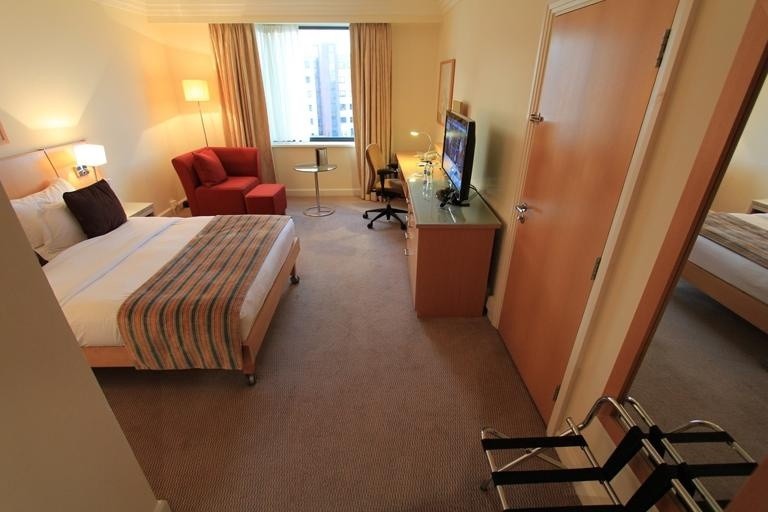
[595,0,768,512]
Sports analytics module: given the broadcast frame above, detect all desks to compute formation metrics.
[293,163,337,217]
[395,149,502,320]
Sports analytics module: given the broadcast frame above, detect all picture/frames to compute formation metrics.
[437,59,456,127]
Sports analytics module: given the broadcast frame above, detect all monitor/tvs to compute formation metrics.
[440,110,475,207]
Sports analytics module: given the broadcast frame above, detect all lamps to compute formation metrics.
[410,131,438,162]
[73,144,108,183]
[181,79,209,147]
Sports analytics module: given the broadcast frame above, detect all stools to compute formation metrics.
[244,183,287,215]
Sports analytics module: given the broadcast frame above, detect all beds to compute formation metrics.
[0,138,301,385]
[680,212,768,334]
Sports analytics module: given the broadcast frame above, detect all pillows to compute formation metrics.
[192,148,228,188]
[63,179,127,239]
[9,177,87,262]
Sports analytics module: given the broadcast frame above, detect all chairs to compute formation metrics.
[363,144,408,230]
[171,147,263,217]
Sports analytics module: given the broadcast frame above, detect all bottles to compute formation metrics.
[423,161,433,184]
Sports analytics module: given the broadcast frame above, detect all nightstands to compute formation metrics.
[123,201,156,217]
[746,199,768,214]
